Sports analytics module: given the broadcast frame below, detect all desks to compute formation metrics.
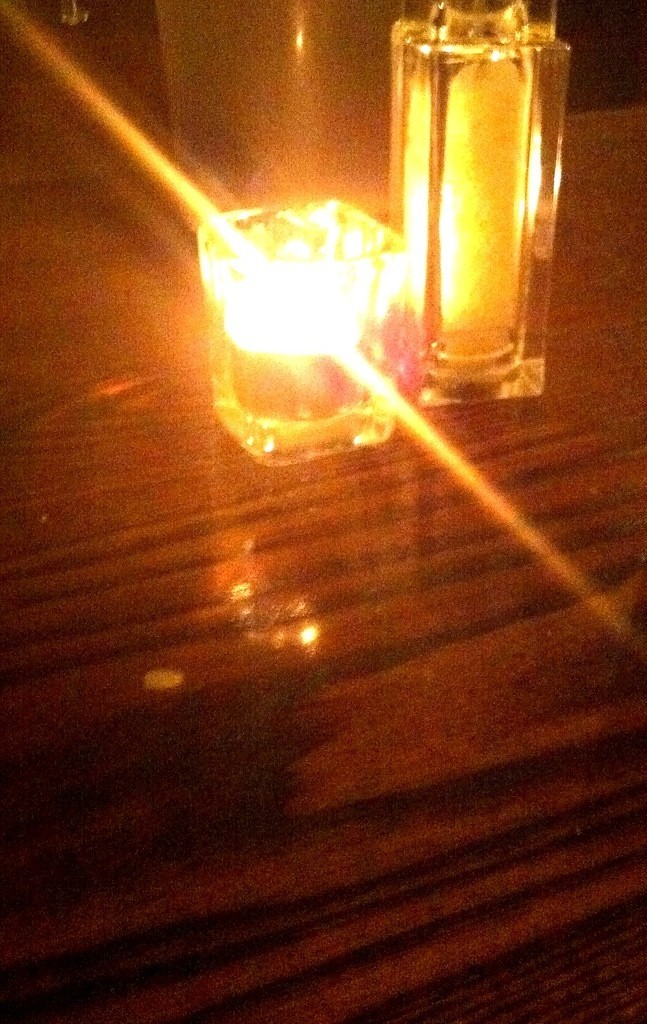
[0,100,647,1024]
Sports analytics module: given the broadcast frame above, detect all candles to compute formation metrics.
[198,200,410,465]
[440,53,526,340]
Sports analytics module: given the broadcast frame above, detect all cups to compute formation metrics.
[201,199,403,465]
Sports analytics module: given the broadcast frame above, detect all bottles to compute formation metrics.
[385,0,572,407]
